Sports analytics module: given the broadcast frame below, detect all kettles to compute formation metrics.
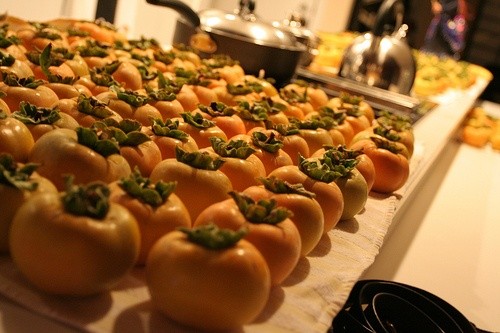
[338,0,417,95]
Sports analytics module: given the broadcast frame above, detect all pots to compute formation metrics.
[145,0,307,91]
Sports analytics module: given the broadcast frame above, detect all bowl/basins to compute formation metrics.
[333,278,476,333]
